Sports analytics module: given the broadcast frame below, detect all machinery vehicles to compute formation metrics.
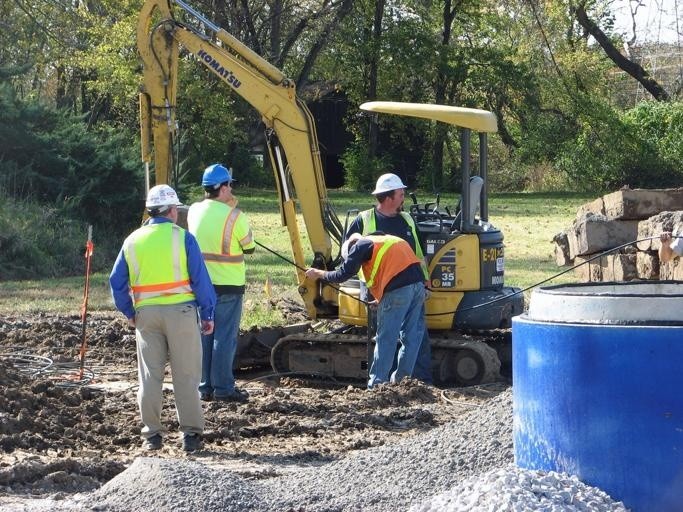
[131,0,523,384]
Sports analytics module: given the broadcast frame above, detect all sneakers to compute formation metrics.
[199,388,250,404]
[182,432,203,452]
[141,434,162,451]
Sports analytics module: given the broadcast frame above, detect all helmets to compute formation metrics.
[371,173,407,195]
[144,184,183,208]
[341,232,363,261]
[201,163,234,186]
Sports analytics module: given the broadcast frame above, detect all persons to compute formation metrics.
[347,173,434,386]
[110,184,217,450]
[305,232,425,389]
[187,164,256,402]
